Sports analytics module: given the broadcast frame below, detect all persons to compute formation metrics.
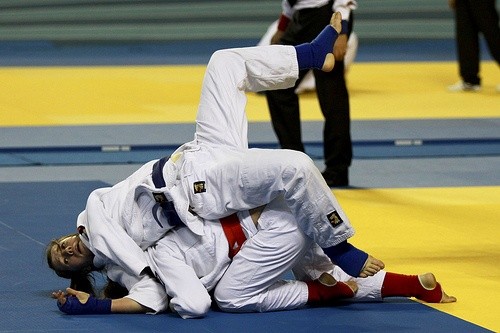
[449,0,500,91]
[46,11,385,316]
[71,195,457,320]
[263,0,357,188]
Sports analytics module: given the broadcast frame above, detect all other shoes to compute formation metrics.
[448,81,480,92]
